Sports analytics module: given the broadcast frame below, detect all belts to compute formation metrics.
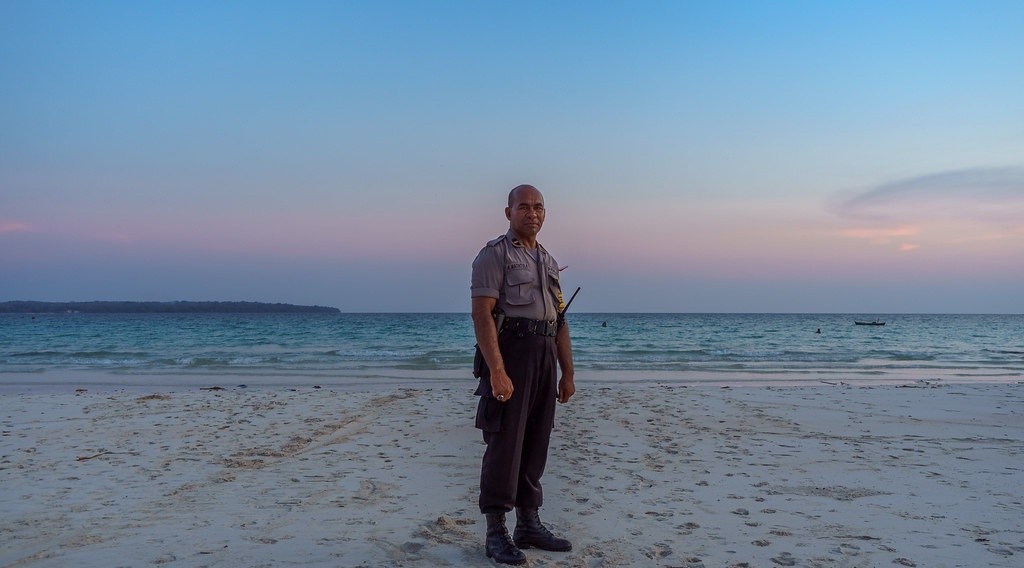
[503,317,558,336]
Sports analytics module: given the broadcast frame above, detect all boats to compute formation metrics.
[854,320,887,326]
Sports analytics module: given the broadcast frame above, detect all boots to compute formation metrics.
[513,507,572,552]
[485,512,526,564]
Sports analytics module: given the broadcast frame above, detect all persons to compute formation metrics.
[470,184,581,565]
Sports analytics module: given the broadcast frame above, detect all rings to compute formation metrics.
[496,394,505,402]
